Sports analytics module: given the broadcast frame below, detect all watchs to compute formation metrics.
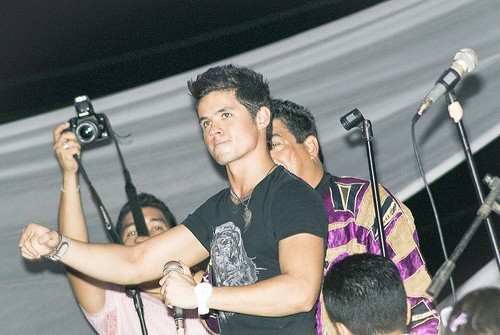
[194,282,213,314]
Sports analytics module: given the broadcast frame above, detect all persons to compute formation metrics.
[322,254,413,335]
[445,287,500,335]
[201,100,441,335]
[18,64,328,335]
[53,122,205,335]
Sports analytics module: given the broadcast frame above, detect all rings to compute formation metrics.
[64,142,68,149]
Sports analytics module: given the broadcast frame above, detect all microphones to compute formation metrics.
[164,261,186,331]
[414,48,478,119]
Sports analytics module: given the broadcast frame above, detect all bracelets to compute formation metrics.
[44,234,70,261]
[60,183,80,193]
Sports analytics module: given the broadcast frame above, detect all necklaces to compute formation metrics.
[230,165,277,218]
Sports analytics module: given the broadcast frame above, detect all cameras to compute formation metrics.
[68,95,111,146]
